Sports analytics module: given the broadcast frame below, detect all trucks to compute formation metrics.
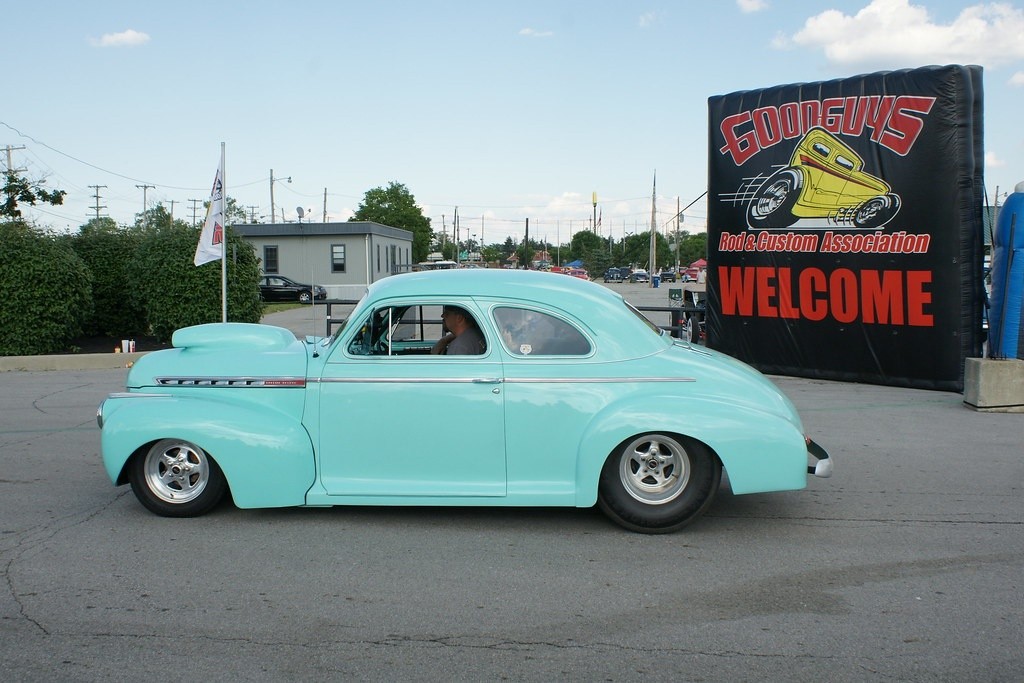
[660,272,677,283]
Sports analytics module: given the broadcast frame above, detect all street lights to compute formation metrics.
[466,228,469,261]
[270,177,293,224]
[472,234,476,260]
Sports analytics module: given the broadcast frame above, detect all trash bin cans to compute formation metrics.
[630,274,636,283]
[653,275,660,288]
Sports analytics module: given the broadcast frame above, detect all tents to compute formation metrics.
[563,260,591,275]
[691,259,707,269]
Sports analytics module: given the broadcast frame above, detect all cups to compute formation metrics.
[121,340,129,353]
[129,341,136,353]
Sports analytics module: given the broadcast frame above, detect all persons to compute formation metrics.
[428,306,487,357]
[567,268,571,275]
[584,269,588,275]
[696,265,706,285]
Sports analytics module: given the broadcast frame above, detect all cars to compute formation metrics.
[97,267,833,536]
[604,268,623,283]
[682,268,698,283]
[680,267,688,276]
[565,269,588,280]
[259,275,327,305]
[630,269,649,283]
[667,283,707,344]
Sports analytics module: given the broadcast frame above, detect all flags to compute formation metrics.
[193,148,222,266]
